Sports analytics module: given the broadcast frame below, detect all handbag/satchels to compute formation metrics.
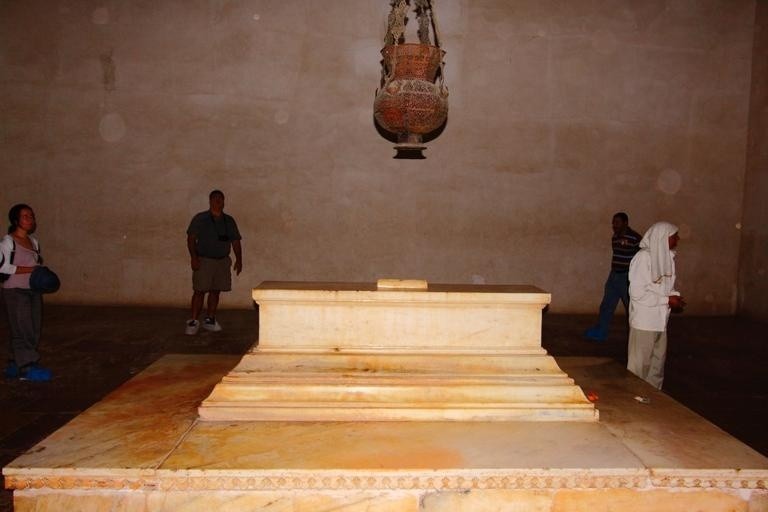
[0,252,15,283]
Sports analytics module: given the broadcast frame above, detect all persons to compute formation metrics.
[184,191,242,336]
[0,204,61,382]
[585,213,643,337]
[626,221,688,391]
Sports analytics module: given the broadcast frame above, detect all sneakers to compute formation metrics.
[19,362,52,383]
[6,359,18,378]
[202,316,222,331]
[580,328,606,341]
[185,319,201,336]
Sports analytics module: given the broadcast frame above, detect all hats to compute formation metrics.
[30,266,61,294]
